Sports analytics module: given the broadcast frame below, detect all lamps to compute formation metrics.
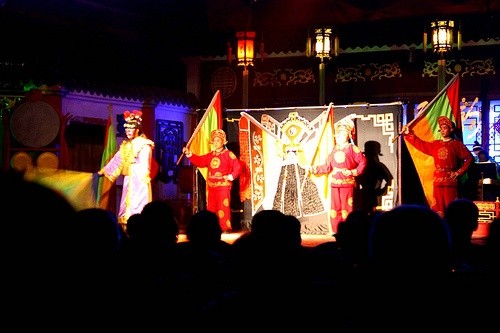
[305,24,340,105]
[423,15,462,93]
[226,30,264,108]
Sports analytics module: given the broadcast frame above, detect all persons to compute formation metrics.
[98,110,153,228]
[402,116,473,218]
[308,120,366,236]
[470,142,500,180]
[183,129,242,232]
[0,201,500,332]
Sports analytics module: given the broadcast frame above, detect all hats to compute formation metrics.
[124,110,143,128]
[208,129,228,145]
[334,123,352,139]
[438,116,455,133]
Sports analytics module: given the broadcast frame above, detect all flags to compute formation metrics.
[96,108,118,215]
[311,105,336,213]
[188,90,222,183]
[403,78,463,214]
